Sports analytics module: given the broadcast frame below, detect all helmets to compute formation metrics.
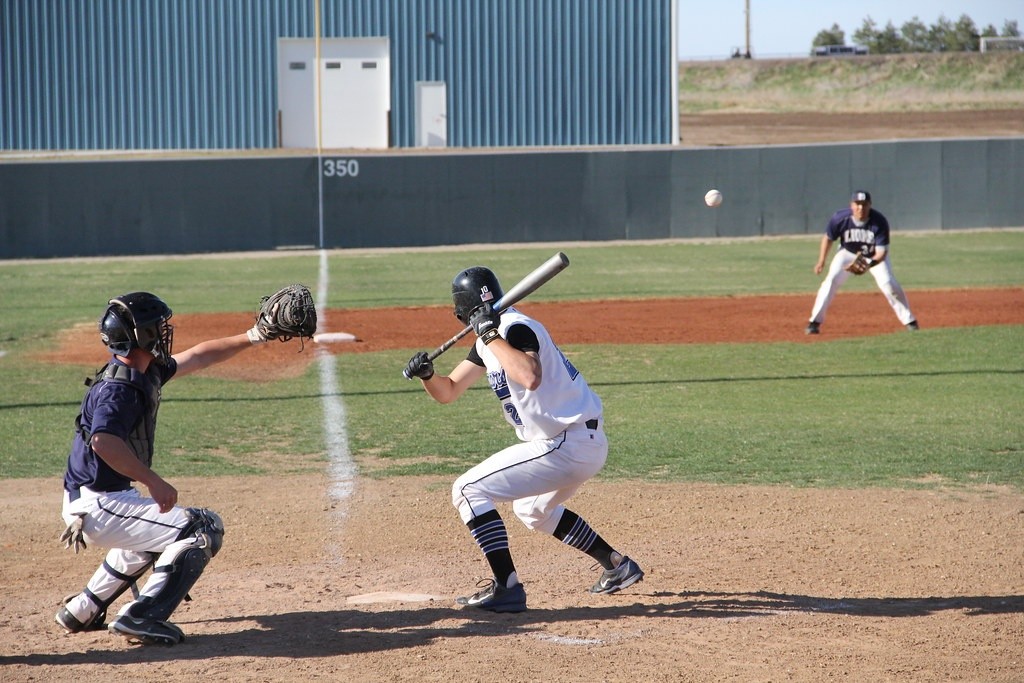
[96,291,172,370]
[451,266,505,327]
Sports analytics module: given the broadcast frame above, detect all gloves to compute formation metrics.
[405,352,434,380]
[469,302,501,346]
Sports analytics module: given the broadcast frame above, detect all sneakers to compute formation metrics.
[583,556,645,595]
[454,578,527,613]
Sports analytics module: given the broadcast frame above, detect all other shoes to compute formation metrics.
[805,325,819,334]
[906,321,919,331]
[54,606,84,634]
[107,615,181,648]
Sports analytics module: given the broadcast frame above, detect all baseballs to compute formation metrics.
[705,189,723,207]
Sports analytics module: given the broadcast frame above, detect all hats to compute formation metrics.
[850,190,871,202]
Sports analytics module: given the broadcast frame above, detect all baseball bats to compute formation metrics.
[401,251,572,381]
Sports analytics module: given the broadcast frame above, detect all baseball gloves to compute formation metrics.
[844,251,870,274]
[255,284,318,345]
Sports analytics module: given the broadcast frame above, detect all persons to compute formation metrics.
[53,290,320,642]
[406,270,646,614]
[804,189,920,333]
